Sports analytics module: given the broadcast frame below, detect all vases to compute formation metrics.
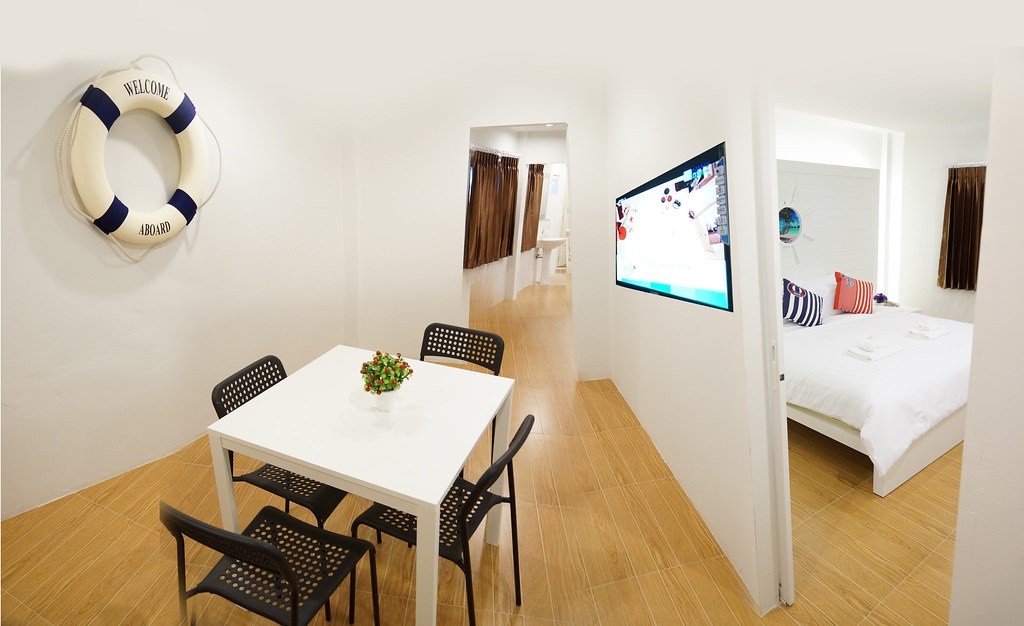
[375,389,400,411]
[876,302,885,307]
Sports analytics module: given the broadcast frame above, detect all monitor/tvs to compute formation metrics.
[616,140,734,313]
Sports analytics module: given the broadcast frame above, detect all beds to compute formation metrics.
[784,282,974,498]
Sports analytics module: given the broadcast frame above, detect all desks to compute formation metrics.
[209,343,516,626]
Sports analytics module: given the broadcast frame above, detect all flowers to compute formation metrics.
[872,292,888,303]
[362,351,414,397]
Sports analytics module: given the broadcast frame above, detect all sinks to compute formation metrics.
[536,236,568,251]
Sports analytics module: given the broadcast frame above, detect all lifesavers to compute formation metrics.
[64,64,211,252]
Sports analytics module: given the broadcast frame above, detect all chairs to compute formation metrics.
[160,499,380,626]
[212,354,348,621]
[345,412,536,626]
[373,322,505,549]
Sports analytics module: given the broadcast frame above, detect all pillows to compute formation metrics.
[832,270,874,314]
[782,278,825,326]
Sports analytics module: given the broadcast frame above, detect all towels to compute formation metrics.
[842,317,953,364]
